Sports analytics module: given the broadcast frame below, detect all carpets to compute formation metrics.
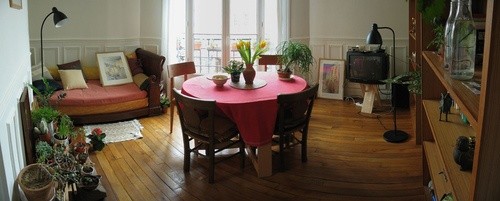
[84,119,143,144]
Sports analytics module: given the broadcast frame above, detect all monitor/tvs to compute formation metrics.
[346,51,389,84]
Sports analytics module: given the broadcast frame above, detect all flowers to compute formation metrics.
[237,41,265,64]
[86,128,106,151]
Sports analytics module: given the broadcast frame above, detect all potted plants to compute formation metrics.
[18,78,101,201]
[224,60,244,82]
[277,41,315,78]
[425,21,474,61]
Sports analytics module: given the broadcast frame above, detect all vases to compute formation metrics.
[243,64,256,85]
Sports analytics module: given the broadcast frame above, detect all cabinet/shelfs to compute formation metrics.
[409,0,500,201]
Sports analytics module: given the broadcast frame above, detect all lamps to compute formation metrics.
[40,7,68,77]
[10,0,22,9]
[366,23,408,143]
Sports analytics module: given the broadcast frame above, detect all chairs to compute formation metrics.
[167,55,319,183]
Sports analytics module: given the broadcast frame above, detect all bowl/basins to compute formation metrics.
[212,75,227,86]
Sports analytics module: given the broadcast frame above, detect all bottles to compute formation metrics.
[443,0,458,72]
[450,0,477,80]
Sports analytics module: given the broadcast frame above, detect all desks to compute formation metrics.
[182,72,307,176]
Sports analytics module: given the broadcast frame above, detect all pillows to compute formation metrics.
[58,69,89,90]
[57,60,88,82]
[32,79,63,96]
[134,73,150,90]
[31,63,53,81]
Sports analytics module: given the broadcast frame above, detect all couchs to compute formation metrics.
[32,48,166,127]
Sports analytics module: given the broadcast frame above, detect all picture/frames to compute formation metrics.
[96,51,134,86]
[316,58,346,100]
[20,87,34,165]
